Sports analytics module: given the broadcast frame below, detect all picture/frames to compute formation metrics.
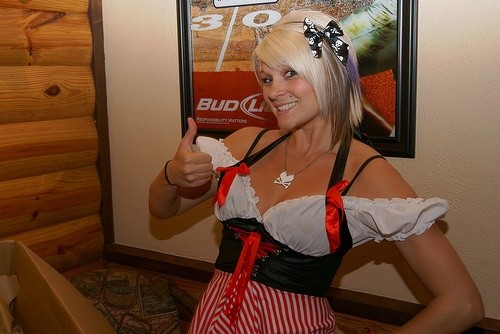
[176,0,418,159]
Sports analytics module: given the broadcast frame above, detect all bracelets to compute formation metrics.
[164,159,178,190]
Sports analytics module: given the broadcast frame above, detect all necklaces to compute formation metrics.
[272,135,334,189]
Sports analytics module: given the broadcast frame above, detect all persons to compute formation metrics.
[149,10,486,333]
